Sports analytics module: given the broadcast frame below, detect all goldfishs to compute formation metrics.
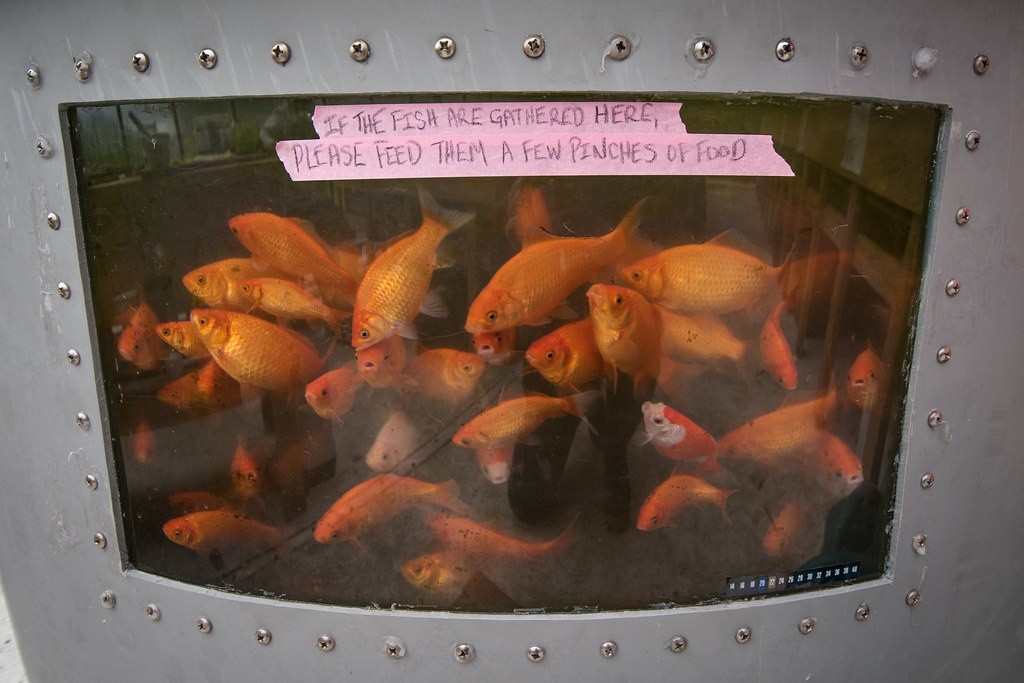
[115,183,890,602]
